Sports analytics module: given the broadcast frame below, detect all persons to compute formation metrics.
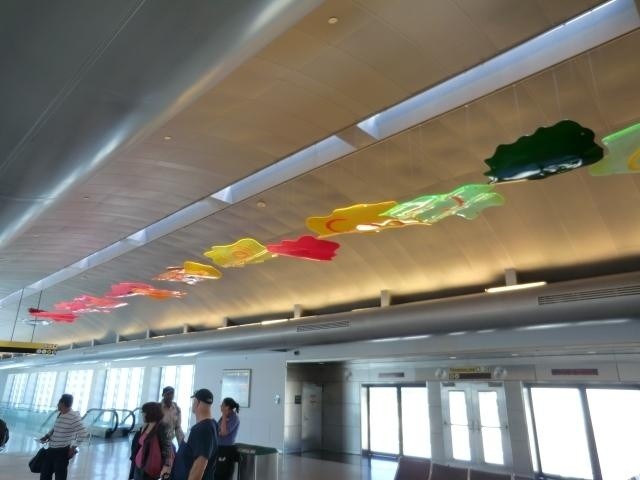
[169,389,219,480]
[215,398,240,480]
[128,401,175,480]
[37,394,88,480]
[160,386,186,451]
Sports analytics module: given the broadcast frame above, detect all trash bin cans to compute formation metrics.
[235,443,282,480]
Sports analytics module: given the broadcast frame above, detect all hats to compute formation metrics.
[162,386,175,394]
[190,388,213,404]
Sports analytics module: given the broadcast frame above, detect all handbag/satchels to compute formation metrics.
[143,435,176,477]
[28,448,46,473]
[217,450,241,462]
[169,438,211,480]
